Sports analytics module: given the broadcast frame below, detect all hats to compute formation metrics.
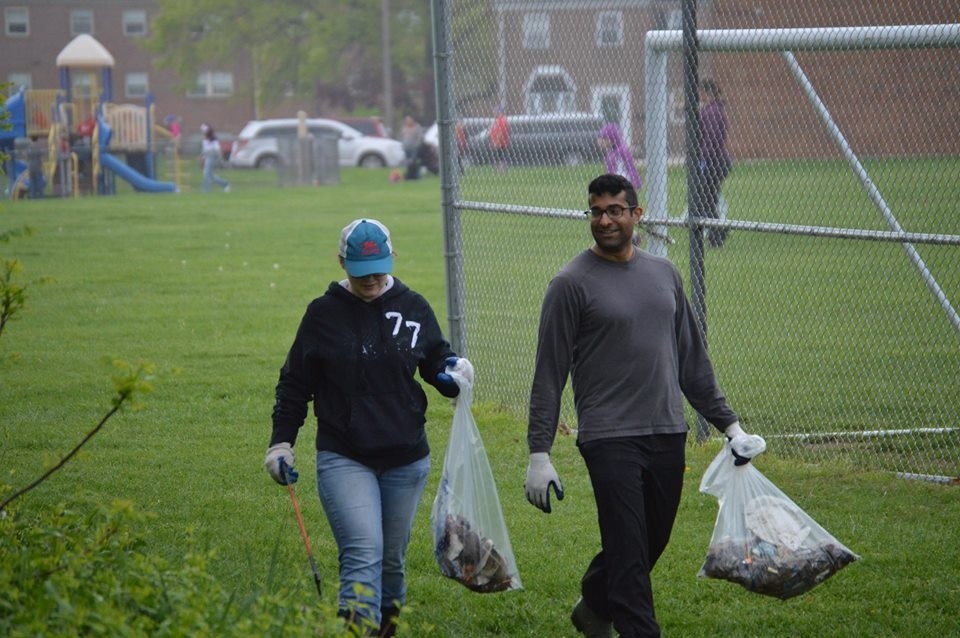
[338,218,395,277]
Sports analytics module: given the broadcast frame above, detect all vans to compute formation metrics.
[424,114,609,172]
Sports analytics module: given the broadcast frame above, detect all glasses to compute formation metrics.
[583,203,637,220]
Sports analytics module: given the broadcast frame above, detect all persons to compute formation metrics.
[524,174,754,638]
[455,116,469,173]
[201,121,229,191]
[698,79,731,234]
[599,124,641,245]
[404,114,422,180]
[265,219,462,638]
[491,106,511,176]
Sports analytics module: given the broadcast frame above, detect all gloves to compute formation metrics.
[436,356,470,391]
[523,451,571,515]
[721,422,758,464]
[263,442,303,483]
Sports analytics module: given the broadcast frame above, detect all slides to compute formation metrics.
[0,138,48,199]
[100,153,176,192]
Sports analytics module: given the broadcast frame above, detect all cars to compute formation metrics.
[201,118,390,166]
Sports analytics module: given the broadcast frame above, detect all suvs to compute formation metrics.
[228,117,406,170]
